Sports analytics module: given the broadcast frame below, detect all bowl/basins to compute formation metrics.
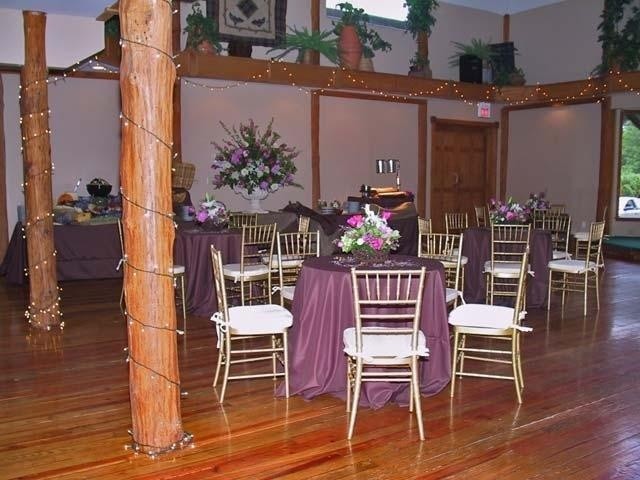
[86,184,112,197]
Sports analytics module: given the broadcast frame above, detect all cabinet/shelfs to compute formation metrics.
[348,191,413,215]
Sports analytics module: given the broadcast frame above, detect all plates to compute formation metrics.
[314,199,364,216]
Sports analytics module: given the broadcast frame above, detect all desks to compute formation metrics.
[0,220,196,285]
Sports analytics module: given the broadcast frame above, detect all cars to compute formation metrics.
[618,194,640,218]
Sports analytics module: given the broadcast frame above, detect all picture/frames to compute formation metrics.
[206,0,287,49]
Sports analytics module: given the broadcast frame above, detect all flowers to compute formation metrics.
[211,119,304,194]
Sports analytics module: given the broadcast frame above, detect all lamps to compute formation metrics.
[376,159,401,192]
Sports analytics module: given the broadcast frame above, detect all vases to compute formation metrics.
[241,189,270,214]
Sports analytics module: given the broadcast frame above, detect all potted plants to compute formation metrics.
[496,66,525,85]
[447,39,499,83]
[331,2,390,69]
[265,25,351,65]
[403,0,439,79]
[183,3,222,54]
